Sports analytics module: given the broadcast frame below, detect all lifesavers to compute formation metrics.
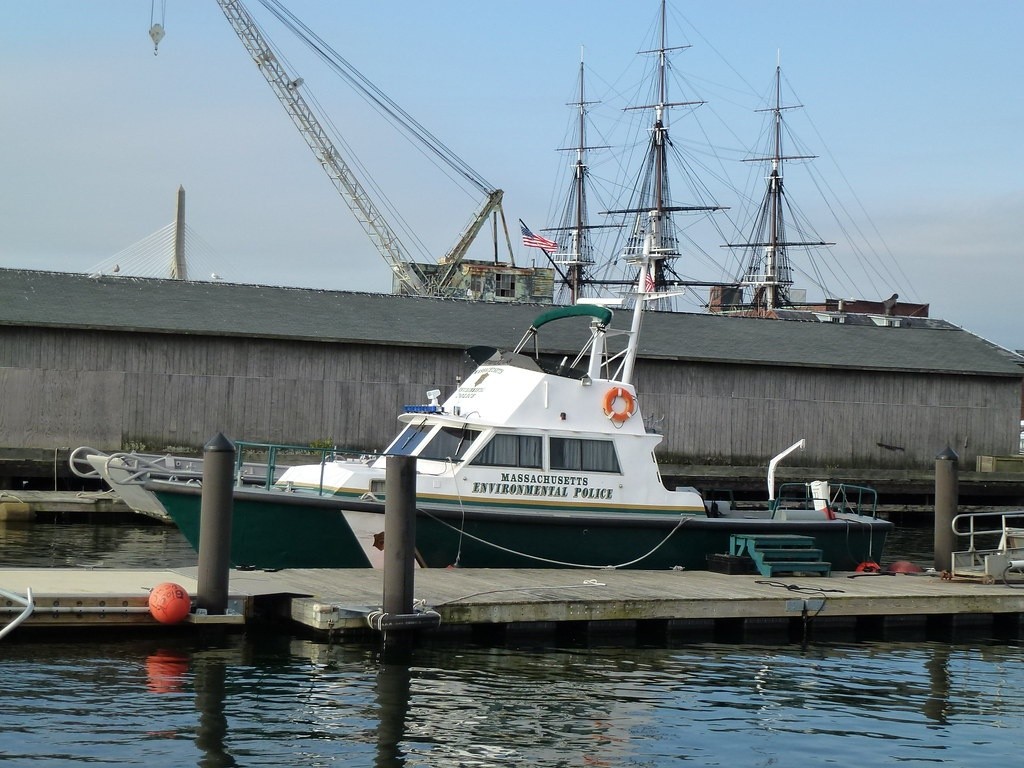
[601,385,636,424]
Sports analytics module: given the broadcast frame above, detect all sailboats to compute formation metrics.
[0,0,1024,521]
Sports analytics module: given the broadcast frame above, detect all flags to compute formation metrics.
[520,225,558,252]
[645,263,655,292]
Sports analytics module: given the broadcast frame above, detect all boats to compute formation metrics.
[71,215,896,575]
